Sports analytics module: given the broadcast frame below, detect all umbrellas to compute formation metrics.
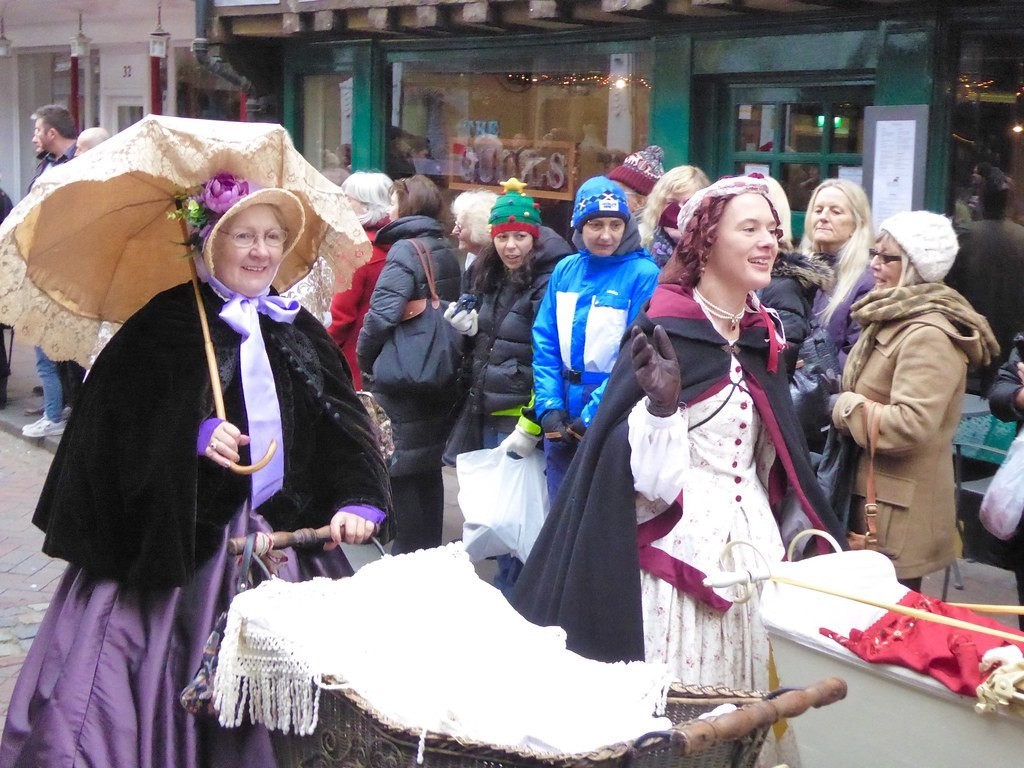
[0,114,373,474]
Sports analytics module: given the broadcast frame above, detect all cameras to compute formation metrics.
[451,293,478,318]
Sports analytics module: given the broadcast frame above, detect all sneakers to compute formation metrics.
[21,386,72,438]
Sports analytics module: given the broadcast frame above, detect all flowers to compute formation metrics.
[169,170,250,257]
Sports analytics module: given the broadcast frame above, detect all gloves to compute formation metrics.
[502,430,538,457]
[630,326,682,417]
[443,301,472,331]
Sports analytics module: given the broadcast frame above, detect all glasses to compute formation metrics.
[218,229,289,246]
[868,248,902,264]
[454,221,463,233]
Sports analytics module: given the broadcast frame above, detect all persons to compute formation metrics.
[0,173,398,768]
[942,161,1024,379]
[754,162,1024,631]
[502,173,851,768]
[321,126,566,190]
[321,145,708,559]
[0,105,109,436]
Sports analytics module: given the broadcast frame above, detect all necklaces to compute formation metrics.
[693,287,744,331]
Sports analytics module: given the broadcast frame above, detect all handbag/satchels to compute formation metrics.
[180,532,274,715]
[456,444,550,566]
[371,299,463,394]
[847,531,880,551]
[788,329,841,378]
[978,428,1023,540]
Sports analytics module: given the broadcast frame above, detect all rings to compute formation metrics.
[210,444,215,448]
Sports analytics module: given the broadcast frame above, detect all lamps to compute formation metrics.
[147,1,171,59]
[69,11,92,58]
[0,15,14,58]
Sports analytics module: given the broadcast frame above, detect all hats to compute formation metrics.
[189,188,305,277]
[486,177,541,239]
[678,174,783,240]
[606,145,664,195]
[881,211,960,283]
[572,177,631,233]
